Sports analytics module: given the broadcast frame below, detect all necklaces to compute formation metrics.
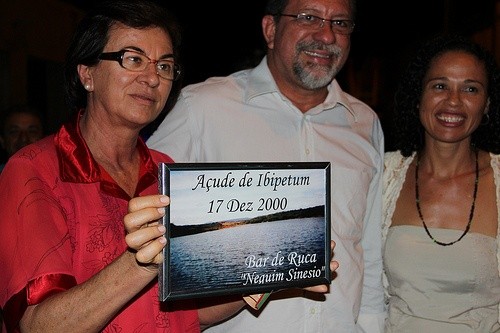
[415,146,479,247]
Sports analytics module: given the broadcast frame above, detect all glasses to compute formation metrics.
[99,49,182,83]
[278,12,355,36]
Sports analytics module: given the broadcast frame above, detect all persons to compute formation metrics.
[0,0,338,333]
[144,1,385,333]
[0,106,43,174]
[384,34,499,333]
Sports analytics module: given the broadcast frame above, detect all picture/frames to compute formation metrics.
[158,161,332,306]
